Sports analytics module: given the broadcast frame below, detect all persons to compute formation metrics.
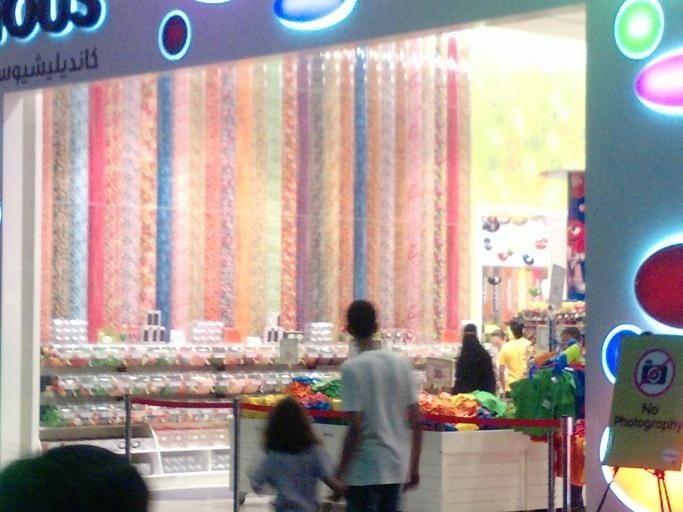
[334,300,421,512]
[451,320,585,401]
[248,395,347,511]
[0,444,147,511]
